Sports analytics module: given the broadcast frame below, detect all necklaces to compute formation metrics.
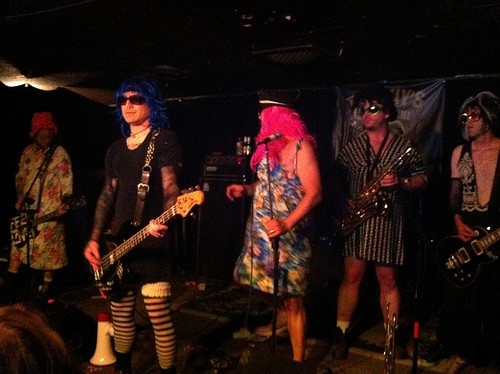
[130,125,151,138]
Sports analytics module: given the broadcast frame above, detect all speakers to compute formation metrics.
[195,178,256,285]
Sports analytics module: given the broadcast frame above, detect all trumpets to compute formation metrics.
[382,301,397,374]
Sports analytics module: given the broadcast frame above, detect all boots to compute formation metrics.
[113,351,131,374]
[160,366,175,374]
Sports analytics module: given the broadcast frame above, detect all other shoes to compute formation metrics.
[254,319,286,335]
[331,326,346,359]
[392,328,403,359]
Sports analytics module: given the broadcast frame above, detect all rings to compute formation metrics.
[270,229,274,232]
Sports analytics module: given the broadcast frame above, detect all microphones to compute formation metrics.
[44,134,60,151]
[259,132,281,145]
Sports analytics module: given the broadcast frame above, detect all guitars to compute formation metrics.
[10,193,88,250]
[435,225,499,288]
[87,184,206,300]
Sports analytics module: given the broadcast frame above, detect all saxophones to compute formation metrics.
[335,138,412,237]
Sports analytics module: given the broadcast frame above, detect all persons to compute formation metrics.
[425,91,500,362]
[4,112,73,303]
[325,83,428,362]
[226,90,323,374]
[0,301,85,374]
[83,77,182,374]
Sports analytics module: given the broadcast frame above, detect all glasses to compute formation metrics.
[459,113,483,125]
[359,104,387,117]
[120,95,148,106]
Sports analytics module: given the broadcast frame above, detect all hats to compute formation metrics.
[28,112,58,135]
[255,86,301,108]
